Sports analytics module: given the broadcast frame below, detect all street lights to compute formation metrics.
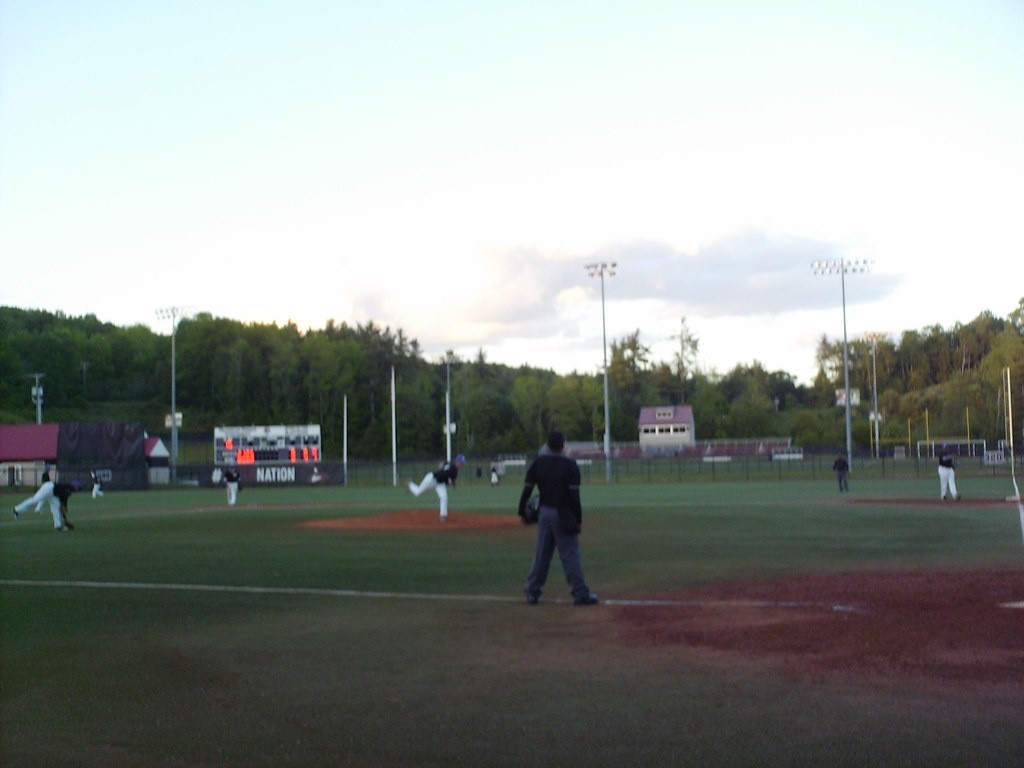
[861,331,887,458]
[583,261,617,455]
[154,305,197,487]
[810,257,875,474]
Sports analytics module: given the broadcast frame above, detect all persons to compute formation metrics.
[938,443,964,502]
[13,463,79,534]
[405,454,466,523]
[518,430,602,605]
[222,460,240,506]
[89,469,104,499]
[831,454,850,493]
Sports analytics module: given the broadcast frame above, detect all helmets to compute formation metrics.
[523,494,541,525]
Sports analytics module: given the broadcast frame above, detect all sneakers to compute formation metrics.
[57,526,68,531]
[11,507,19,519]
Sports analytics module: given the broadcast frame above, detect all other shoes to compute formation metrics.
[440,516,447,521]
[526,593,538,603]
[956,494,962,501]
[574,593,599,605]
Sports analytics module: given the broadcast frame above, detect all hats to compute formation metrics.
[72,481,81,487]
[943,443,951,449]
[455,454,467,464]
